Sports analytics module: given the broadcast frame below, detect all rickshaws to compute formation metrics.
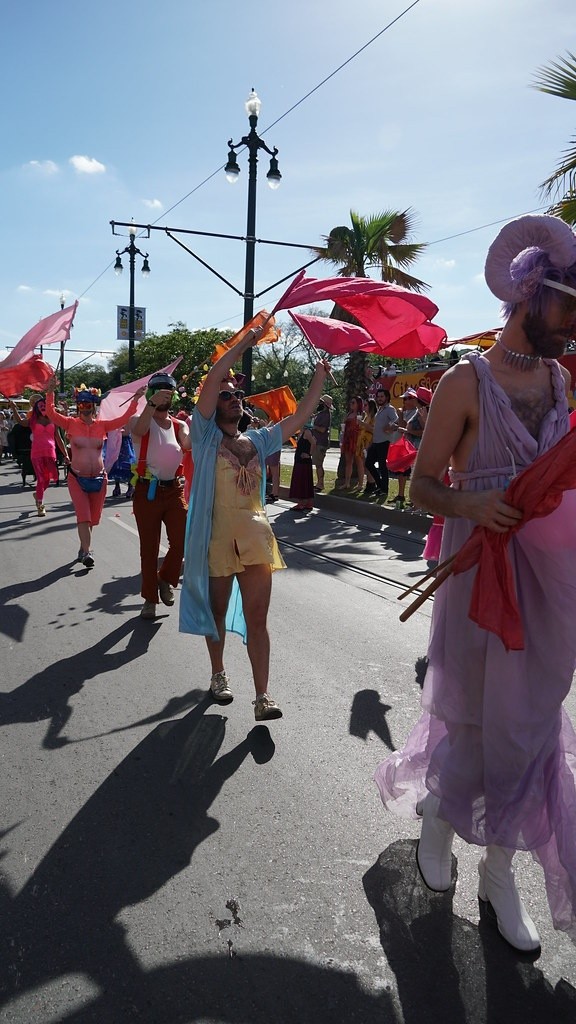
[7,424,69,487]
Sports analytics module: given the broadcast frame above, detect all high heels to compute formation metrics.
[415,788,455,892]
[477,857,542,952]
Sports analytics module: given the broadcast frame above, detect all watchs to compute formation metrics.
[148,400,156,407]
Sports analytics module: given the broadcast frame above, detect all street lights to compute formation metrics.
[38,316,43,354]
[223,86,282,396]
[114,217,151,370]
[59,295,74,393]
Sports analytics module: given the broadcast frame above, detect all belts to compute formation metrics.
[139,477,175,487]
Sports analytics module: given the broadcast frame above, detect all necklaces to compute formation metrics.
[223,432,241,438]
[495,333,538,360]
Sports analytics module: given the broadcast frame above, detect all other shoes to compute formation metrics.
[113,487,121,496]
[126,488,134,498]
[289,478,428,515]
[83,553,94,567]
[78,550,83,561]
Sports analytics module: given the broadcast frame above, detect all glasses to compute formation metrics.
[220,390,245,401]
[78,403,93,410]
[403,397,411,401]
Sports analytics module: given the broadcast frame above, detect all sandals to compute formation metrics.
[265,494,279,501]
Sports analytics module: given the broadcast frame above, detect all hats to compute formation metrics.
[319,394,334,409]
[408,387,432,404]
[147,371,176,390]
[399,388,416,398]
[74,383,101,402]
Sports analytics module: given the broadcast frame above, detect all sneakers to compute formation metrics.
[252,694,283,722]
[37,505,46,516]
[33,493,36,499]
[158,571,174,606]
[141,601,156,618]
[210,671,234,699]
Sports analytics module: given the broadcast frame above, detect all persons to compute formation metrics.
[240,408,281,501]
[45,378,148,566]
[128,372,191,619]
[308,395,336,490]
[289,417,316,511]
[102,428,135,496]
[337,387,433,506]
[180,326,333,720]
[373,214,576,953]
[0,394,70,517]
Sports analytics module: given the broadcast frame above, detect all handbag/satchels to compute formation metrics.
[69,467,105,491]
[386,433,418,472]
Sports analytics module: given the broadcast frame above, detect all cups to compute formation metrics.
[356,415,362,425]
